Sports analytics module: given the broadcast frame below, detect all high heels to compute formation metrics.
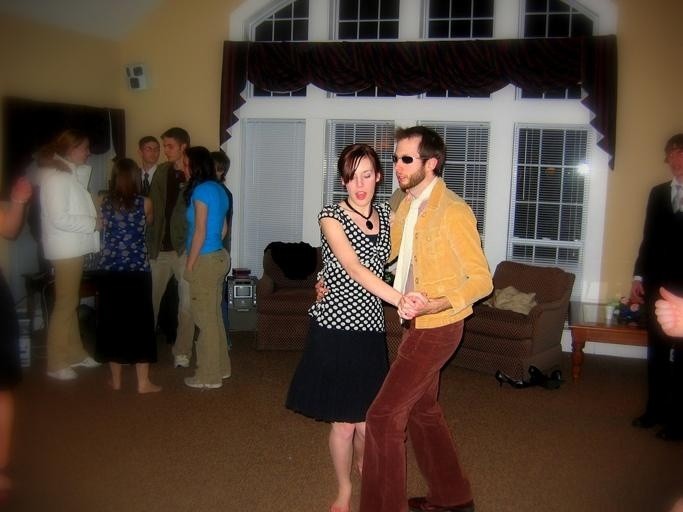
[528,366,560,388]
[494,369,531,388]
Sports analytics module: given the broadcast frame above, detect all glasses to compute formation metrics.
[392,155,428,164]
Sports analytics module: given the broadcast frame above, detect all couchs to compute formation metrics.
[254,242,321,352]
[448,260,577,380]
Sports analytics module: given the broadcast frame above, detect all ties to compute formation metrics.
[673,186,682,213]
[143,172,149,197]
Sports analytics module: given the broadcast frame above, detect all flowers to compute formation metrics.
[613,296,648,326]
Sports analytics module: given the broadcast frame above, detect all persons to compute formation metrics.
[631,133,683,443]
[654,287,683,337]
[285,144,420,512]
[314,126,494,512]
[37,127,233,396]
[0,177,33,500]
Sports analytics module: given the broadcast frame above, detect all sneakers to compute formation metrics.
[45,353,231,389]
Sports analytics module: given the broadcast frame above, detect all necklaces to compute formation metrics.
[345,198,373,230]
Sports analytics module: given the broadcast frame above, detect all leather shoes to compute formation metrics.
[656,424,682,440]
[408,498,477,512]
[633,412,667,428]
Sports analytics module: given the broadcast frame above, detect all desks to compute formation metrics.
[568,300,650,382]
[24,270,100,351]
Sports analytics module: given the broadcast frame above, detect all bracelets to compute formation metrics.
[10,195,28,204]
